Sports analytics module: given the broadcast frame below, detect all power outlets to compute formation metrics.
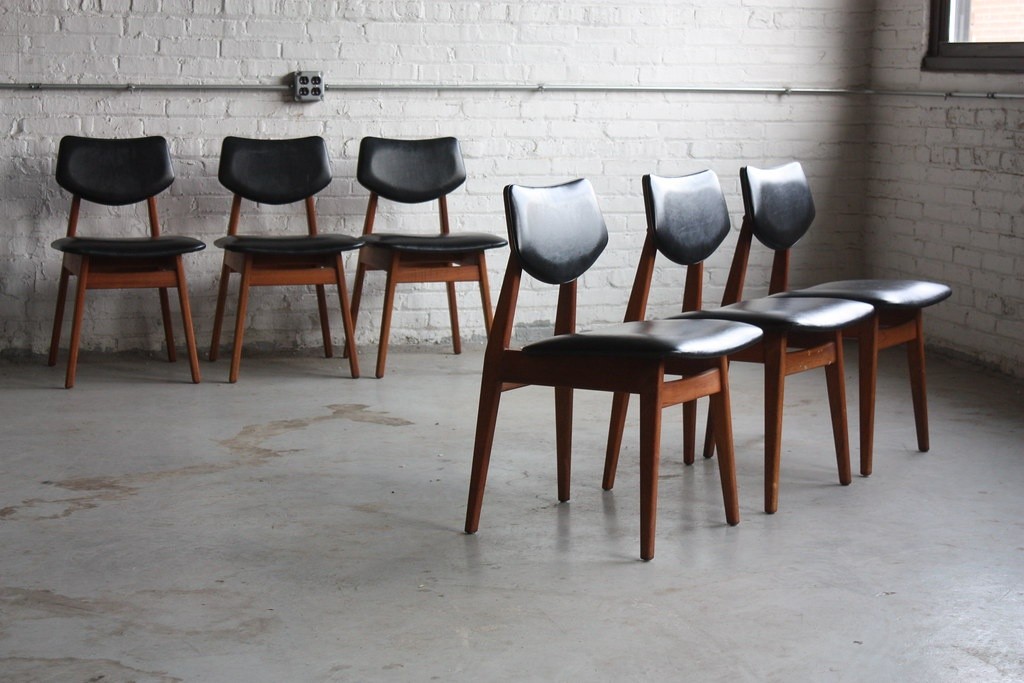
[293,70,324,103]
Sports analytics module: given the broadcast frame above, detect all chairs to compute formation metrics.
[344,136,508,378]
[211,135,362,382]
[703,161,953,463]
[47,134,202,388]
[600,169,876,515]
[463,178,763,561]
